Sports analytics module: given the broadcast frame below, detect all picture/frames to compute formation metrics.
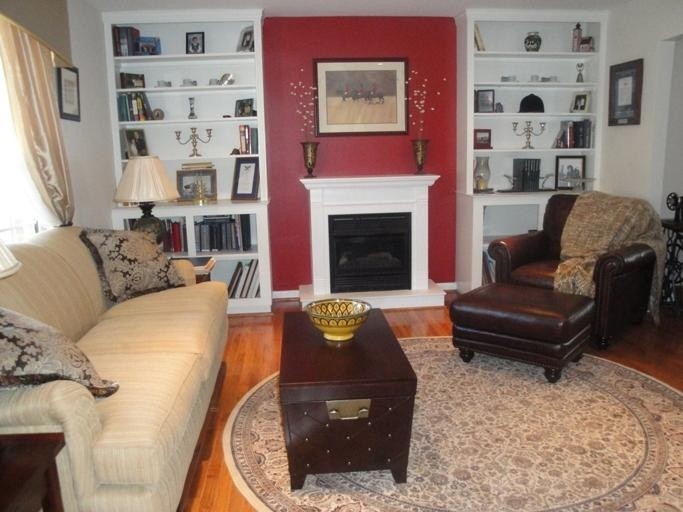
[57,63,81,122]
[607,58,644,126]
[570,91,591,114]
[181,25,254,118]
[311,56,410,136]
[175,157,260,204]
[474,89,495,148]
[554,155,585,191]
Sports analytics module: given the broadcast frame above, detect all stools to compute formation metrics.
[448,282,597,384]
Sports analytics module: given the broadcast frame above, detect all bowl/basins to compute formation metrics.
[305,299,372,340]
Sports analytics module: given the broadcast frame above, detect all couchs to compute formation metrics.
[0,221,230,512]
[487,193,667,351]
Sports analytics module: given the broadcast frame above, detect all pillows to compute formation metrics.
[0,304,120,396]
[79,228,187,305]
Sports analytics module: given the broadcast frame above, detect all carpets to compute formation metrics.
[220,335,683,512]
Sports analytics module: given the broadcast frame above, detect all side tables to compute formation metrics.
[0,429,67,512]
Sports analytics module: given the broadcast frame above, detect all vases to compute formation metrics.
[299,141,321,178]
[472,156,495,194]
[410,139,430,175]
[523,31,542,52]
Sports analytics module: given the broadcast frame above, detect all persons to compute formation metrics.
[129,138,138,154]
[189,36,201,52]
[134,132,146,155]
[566,166,575,187]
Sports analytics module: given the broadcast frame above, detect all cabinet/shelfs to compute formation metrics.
[100,7,272,315]
[454,7,610,291]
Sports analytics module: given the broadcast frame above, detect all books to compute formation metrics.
[160,215,260,298]
[513,158,540,191]
[561,120,590,149]
[113,27,139,56]
[240,125,258,154]
[474,25,485,51]
[117,93,152,122]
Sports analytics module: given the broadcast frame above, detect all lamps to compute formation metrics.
[0,234,22,281]
[111,155,181,253]
